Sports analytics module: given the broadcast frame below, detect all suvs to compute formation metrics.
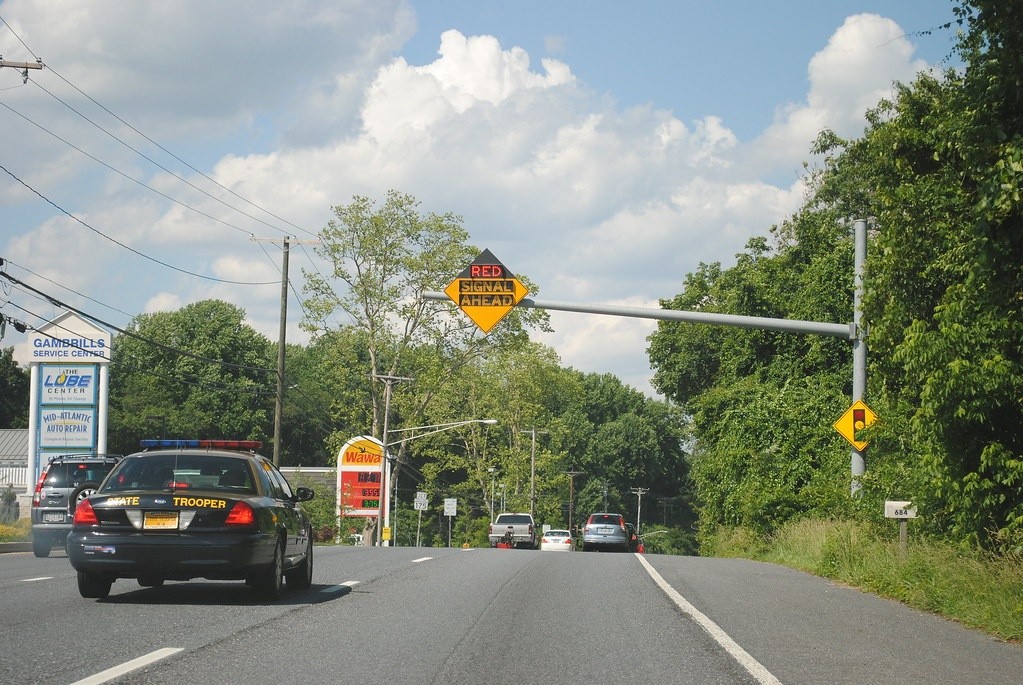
[29,454,124,559]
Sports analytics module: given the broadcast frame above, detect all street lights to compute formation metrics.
[488,467,496,528]
[498,484,506,514]
[519,429,549,520]
[376,420,499,547]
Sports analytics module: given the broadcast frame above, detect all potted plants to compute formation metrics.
[0,483,20,525]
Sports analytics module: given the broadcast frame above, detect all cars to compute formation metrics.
[541,529,574,552]
[625,522,640,554]
[66,440,316,602]
[582,513,630,552]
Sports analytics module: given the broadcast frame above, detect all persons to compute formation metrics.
[201,464,222,476]
[220,469,246,487]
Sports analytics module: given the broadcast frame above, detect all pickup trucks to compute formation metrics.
[488,512,541,550]
